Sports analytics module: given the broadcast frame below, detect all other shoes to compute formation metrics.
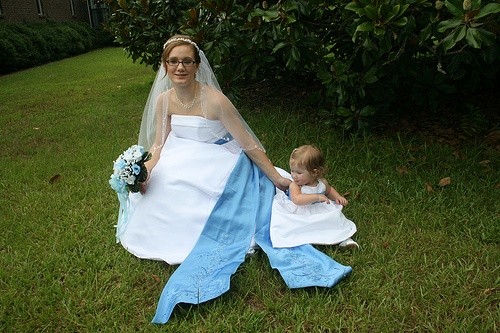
[339,239,359,252]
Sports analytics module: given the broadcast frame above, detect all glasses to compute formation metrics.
[165,59,196,67]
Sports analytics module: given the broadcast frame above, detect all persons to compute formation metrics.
[131,36,293,254]
[269,144,359,253]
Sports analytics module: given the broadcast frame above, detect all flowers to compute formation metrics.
[109,144,152,193]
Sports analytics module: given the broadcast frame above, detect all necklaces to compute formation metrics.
[172,79,199,108]
